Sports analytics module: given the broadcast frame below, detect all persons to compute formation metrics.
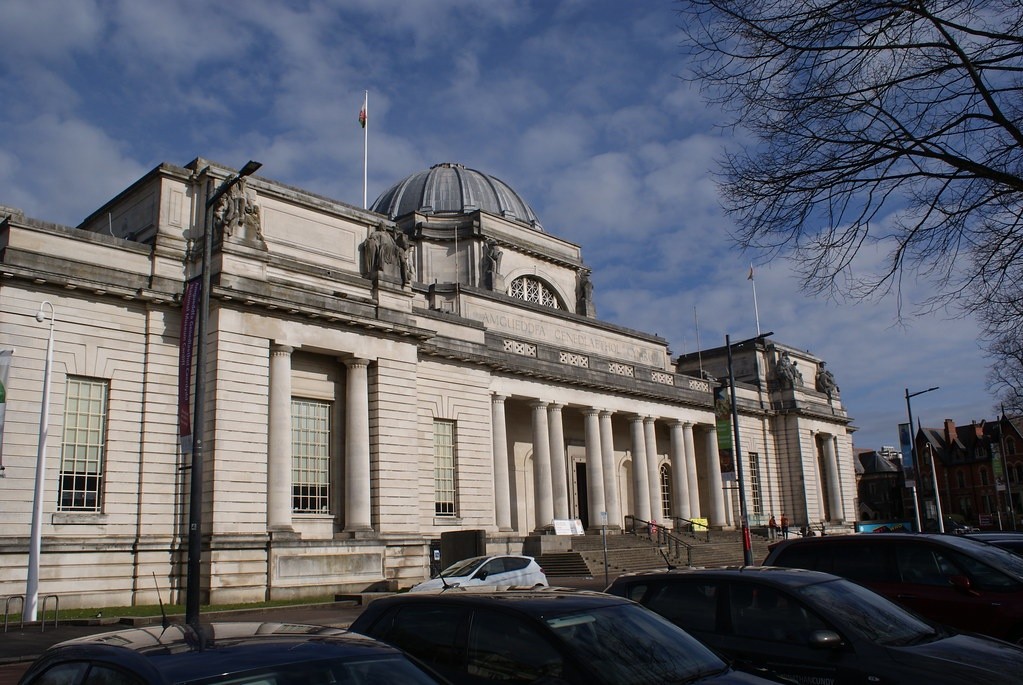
[776,361,804,388]
[816,368,835,393]
[781,514,788,540]
[807,522,816,536]
[769,516,783,536]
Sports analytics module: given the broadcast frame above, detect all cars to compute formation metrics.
[570,567,1023,685]
[347,584,795,685]
[761,534,1023,643]
[407,555,548,594]
[17,619,451,685]
[937,533,1022,583]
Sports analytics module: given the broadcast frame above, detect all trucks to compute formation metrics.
[977,497,1023,530]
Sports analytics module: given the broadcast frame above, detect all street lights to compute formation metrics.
[184,160,264,617]
[905,386,941,532]
[924,441,945,534]
[725,331,775,565]
[1001,415,1023,531]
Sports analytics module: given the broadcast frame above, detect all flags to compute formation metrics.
[359,103,365,128]
